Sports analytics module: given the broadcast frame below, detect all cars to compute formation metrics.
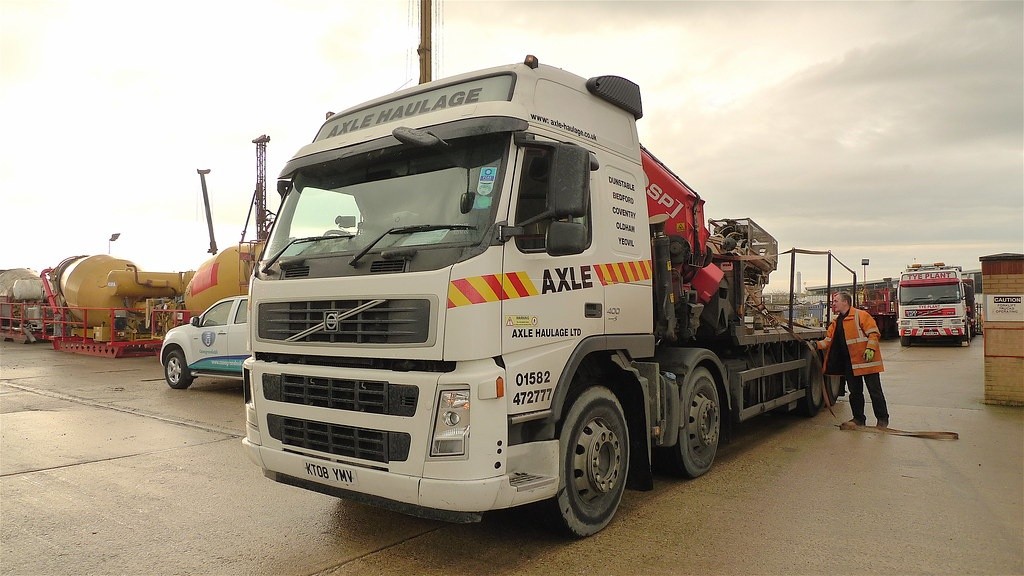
[157,293,249,390]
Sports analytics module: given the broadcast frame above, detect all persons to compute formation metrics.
[806,292,889,428]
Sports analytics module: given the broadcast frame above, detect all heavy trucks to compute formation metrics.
[896,261,975,345]
[240,56,859,539]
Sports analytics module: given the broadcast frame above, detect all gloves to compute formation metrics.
[810,340,817,348]
[863,348,874,362]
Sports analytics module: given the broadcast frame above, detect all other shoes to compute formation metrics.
[876,417,889,429]
[848,416,866,426]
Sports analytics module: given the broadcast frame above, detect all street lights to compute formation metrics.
[862,259,869,305]
[109,233,119,256]
